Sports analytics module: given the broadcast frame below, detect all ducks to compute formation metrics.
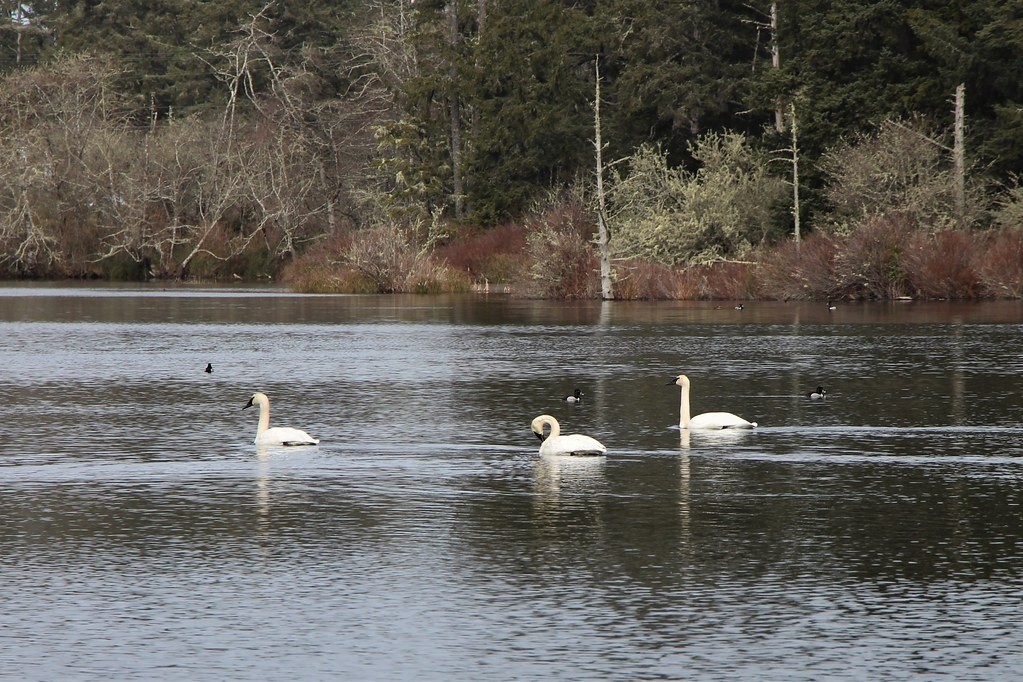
[735,304,744,310]
[825,301,838,310]
[806,387,827,401]
[562,389,584,403]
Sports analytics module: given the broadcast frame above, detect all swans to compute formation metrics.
[664,375,759,430]
[531,414,606,457]
[242,392,320,447]
